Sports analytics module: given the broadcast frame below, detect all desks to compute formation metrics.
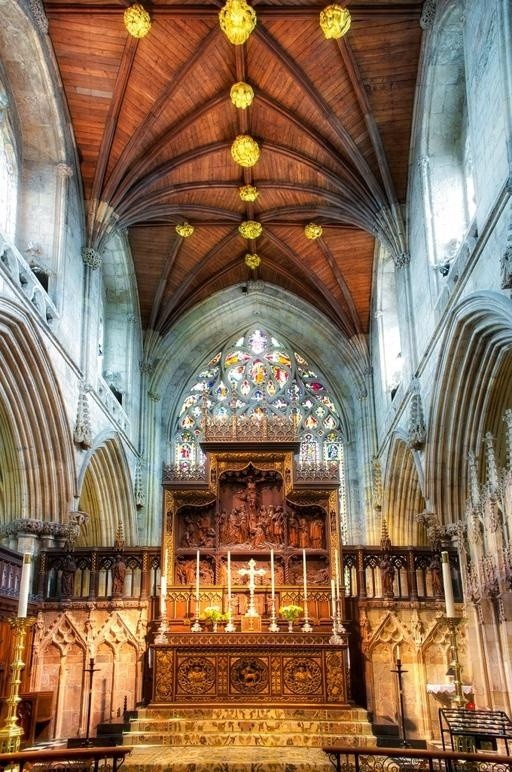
[322,742,512,772]
[1,746,132,771]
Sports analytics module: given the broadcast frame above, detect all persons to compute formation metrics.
[241,475,257,509]
[111,554,126,592]
[181,505,324,548]
[430,555,444,594]
[379,555,395,594]
[60,555,77,596]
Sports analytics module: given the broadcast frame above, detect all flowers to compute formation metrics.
[198,606,232,622]
[279,604,303,623]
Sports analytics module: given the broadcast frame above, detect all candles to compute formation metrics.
[331,579,336,613]
[18,551,33,617]
[90,642,95,658]
[441,550,456,618]
[303,550,308,598]
[334,549,342,599]
[163,545,169,580]
[270,549,274,598]
[396,645,401,661]
[161,576,167,599]
[227,551,231,598]
[196,549,200,600]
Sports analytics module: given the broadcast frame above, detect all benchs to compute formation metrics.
[437,705,511,754]
